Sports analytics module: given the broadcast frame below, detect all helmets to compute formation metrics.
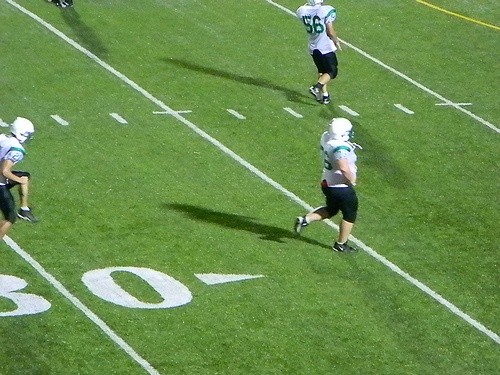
[10,117,34,144]
[328,118,352,142]
[307,0,323,6]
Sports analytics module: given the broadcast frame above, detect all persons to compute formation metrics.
[294,117,363,253]
[296,0,342,104]
[0,117,41,240]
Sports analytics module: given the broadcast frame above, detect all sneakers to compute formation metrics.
[295,215,309,233]
[17,207,39,223]
[332,241,356,253]
[322,95,329,104]
[309,85,323,104]
